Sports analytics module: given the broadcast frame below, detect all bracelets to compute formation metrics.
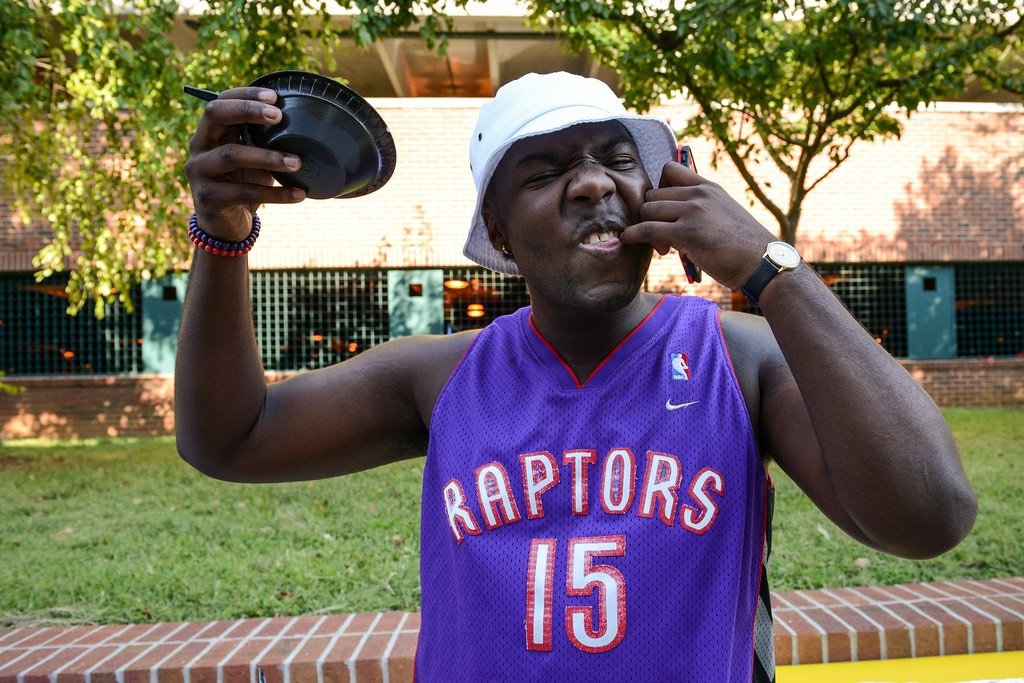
[188,211,262,257]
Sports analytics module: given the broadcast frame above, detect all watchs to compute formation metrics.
[740,241,802,305]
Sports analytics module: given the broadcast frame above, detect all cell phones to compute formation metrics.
[678,145,702,285]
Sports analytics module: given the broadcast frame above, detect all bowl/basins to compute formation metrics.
[241,71,397,200]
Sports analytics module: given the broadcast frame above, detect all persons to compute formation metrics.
[174,70,980,683]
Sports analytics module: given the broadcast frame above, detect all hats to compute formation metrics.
[464,70,679,277]
